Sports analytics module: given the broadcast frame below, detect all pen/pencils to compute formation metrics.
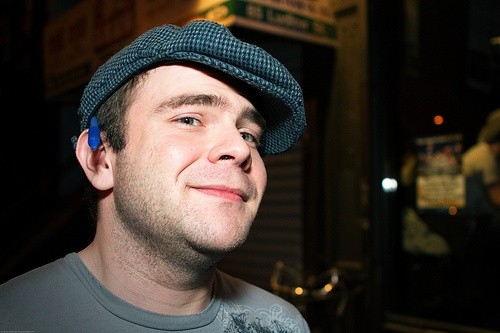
[88,116,101,151]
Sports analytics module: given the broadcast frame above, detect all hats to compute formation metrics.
[78,19,305,156]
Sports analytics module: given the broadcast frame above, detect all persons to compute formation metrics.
[1,20,310,332]
